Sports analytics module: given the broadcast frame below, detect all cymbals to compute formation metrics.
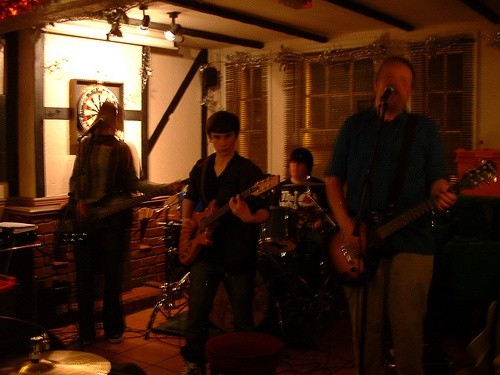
[283,182,326,187]
[0,350,112,375]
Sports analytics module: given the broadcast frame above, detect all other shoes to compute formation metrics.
[108,334,122,343]
[181,361,201,375]
[83,340,92,345]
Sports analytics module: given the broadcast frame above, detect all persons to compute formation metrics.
[323,57,457,375]
[268,147,336,243]
[179,111,273,375]
[69,102,183,344]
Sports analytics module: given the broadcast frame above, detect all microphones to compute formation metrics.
[83,117,106,136]
[380,84,395,105]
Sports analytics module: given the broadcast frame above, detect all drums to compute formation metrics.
[258,206,297,255]
[163,219,192,280]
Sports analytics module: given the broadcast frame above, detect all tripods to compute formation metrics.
[143,197,189,342]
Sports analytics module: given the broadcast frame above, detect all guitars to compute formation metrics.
[323,160,497,284]
[179,174,281,265]
[57,179,189,248]
